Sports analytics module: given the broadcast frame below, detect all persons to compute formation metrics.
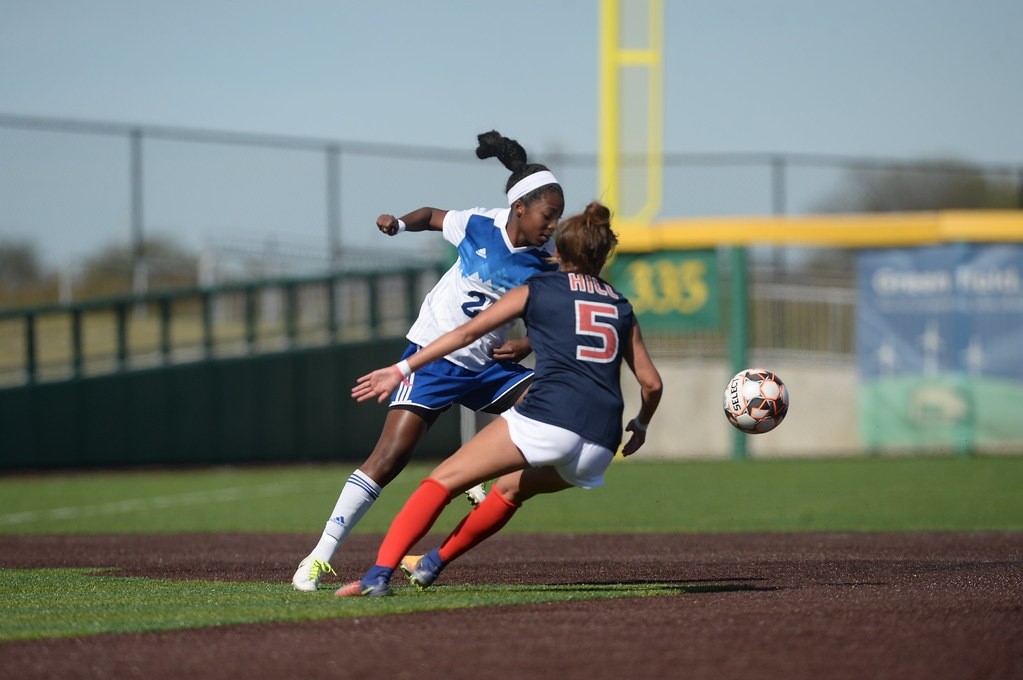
[333,202,663,598]
[292,130,565,592]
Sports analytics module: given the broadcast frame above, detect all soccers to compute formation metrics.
[724,366,789,434]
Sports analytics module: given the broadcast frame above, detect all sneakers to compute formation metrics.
[292,556,337,592]
[400,556,437,591]
[465,485,486,507]
[336,565,393,598]
